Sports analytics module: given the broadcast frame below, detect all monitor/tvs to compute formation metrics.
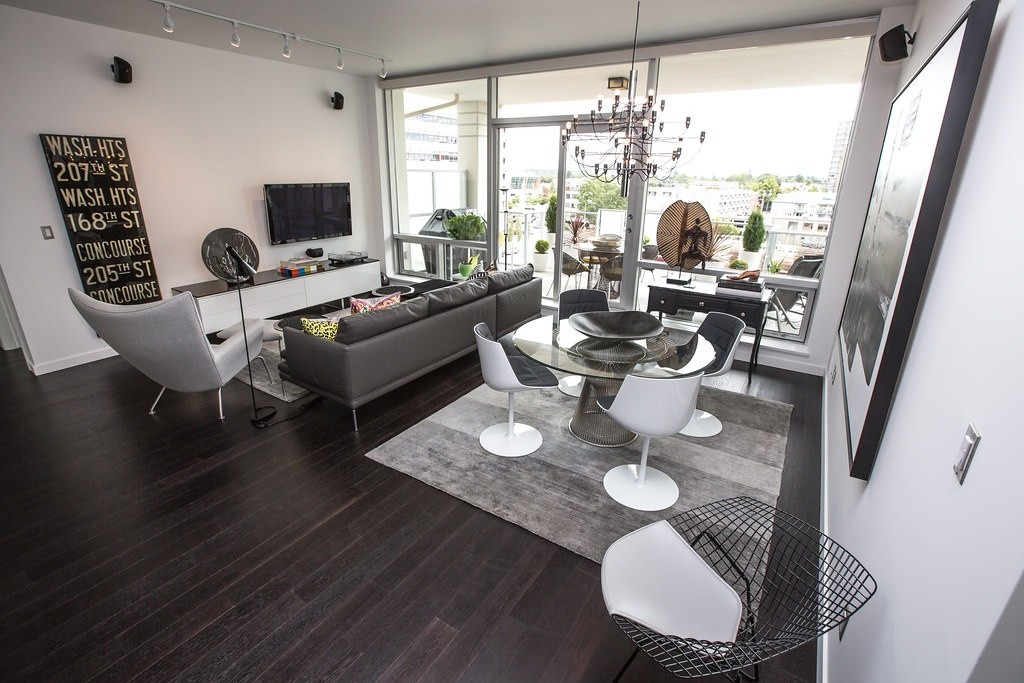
[261,162,353,246]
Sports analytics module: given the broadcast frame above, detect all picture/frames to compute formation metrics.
[836,0,1002,481]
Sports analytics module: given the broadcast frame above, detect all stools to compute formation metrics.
[216,318,283,364]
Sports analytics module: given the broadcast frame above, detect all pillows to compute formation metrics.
[299,292,401,341]
[466,260,486,281]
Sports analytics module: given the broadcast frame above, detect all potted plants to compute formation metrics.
[730,209,786,274]
[444,195,557,277]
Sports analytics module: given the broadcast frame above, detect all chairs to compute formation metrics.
[473,234,877,683]
[67,287,272,420]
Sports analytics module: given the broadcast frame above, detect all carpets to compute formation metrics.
[362,368,794,633]
[235,306,352,404]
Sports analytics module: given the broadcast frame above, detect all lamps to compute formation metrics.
[877,21,917,62]
[226,246,276,425]
[562,0,706,200]
[111,0,394,110]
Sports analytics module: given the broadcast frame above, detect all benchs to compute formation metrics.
[277,263,543,432]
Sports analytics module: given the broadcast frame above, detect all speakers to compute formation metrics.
[879,24,908,62]
[331,92,344,110]
[111,56,132,84]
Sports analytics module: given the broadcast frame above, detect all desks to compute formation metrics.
[571,243,624,299]
[372,285,415,296]
[512,312,679,448]
[273,314,327,330]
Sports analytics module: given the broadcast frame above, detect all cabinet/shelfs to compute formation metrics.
[171,258,381,335]
[649,281,772,385]
